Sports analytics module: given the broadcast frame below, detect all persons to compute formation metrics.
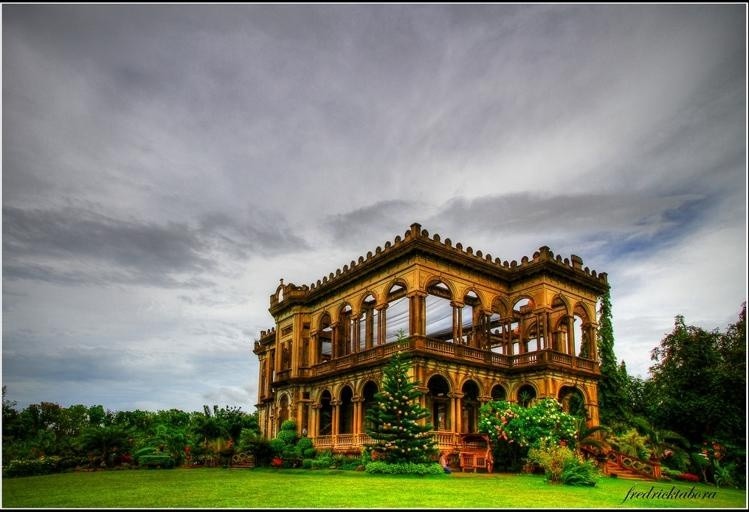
[438,450,452,473]
